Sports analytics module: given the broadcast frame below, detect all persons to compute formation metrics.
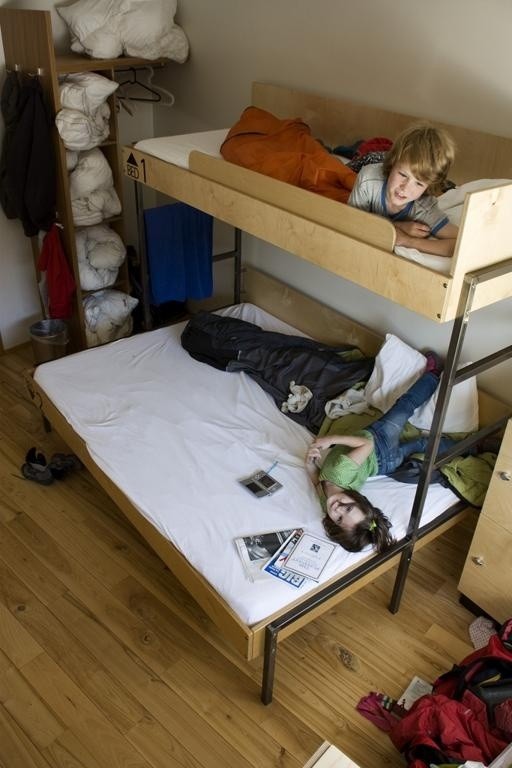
[303,348,482,556]
[316,119,461,257]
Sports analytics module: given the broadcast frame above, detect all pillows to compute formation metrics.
[436,178,512,227]
[363,331,479,433]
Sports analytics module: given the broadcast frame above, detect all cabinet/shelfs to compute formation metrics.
[0,7,169,357]
[457,417,512,633]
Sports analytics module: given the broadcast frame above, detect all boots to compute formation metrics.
[20,444,84,486]
[474,434,502,455]
[423,349,445,378]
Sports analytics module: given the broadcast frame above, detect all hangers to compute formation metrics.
[115,65,175,116]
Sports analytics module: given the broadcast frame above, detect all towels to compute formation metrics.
[143,202,213,304]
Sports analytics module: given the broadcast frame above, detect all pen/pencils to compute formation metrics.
[266,461,278,474]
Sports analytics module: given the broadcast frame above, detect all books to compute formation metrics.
[281,530,338,582]
[261,531,306,589]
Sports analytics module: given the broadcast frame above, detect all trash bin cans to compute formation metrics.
[28,319,69,366]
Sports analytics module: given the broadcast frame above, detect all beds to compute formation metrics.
[25,79,512,705]
